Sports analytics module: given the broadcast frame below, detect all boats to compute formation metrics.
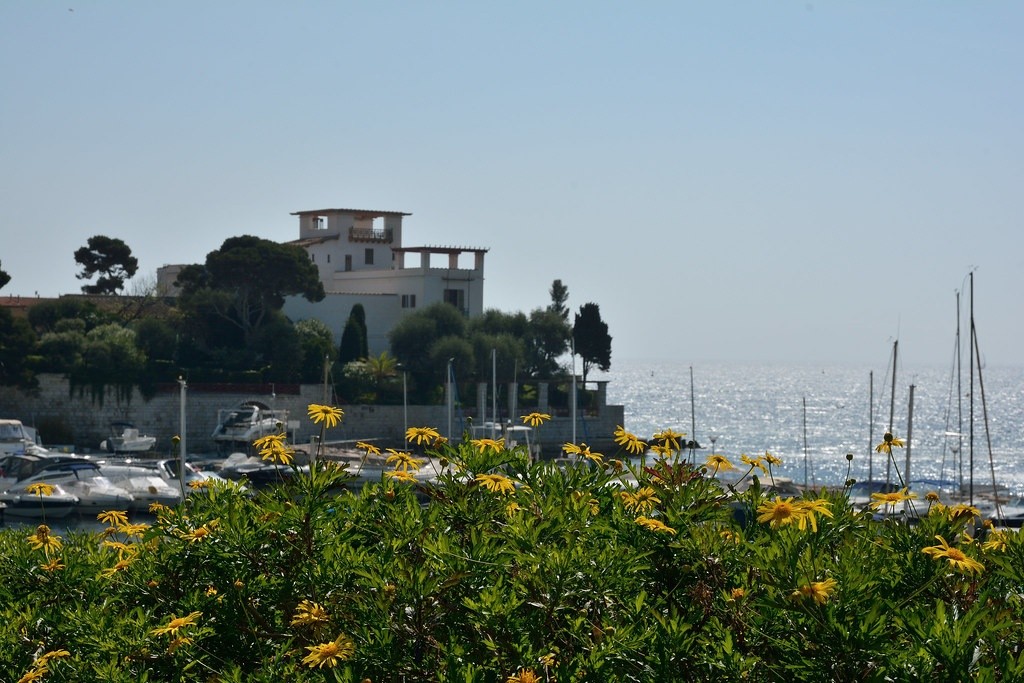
[97,419,156,454]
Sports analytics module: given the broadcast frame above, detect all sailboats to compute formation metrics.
[0,272,1024,526]
